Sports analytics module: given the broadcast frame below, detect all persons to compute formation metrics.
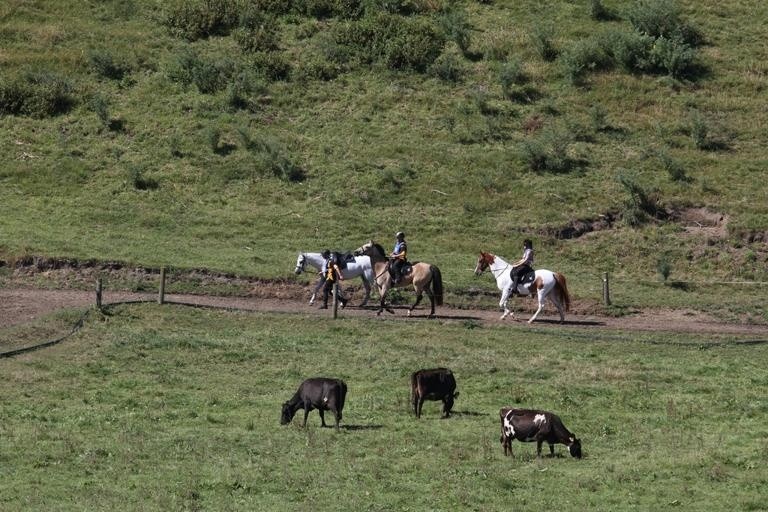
[509,237,534,294]
[316,249,349,311]
[389,230,408,285]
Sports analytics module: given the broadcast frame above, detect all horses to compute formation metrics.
[294,250,377,308]
[353,239,443,319]
[474,249,571,324]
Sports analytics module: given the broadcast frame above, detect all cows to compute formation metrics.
[410,367,460,419]
[499,408,582,460]
[281,377,347,430]
